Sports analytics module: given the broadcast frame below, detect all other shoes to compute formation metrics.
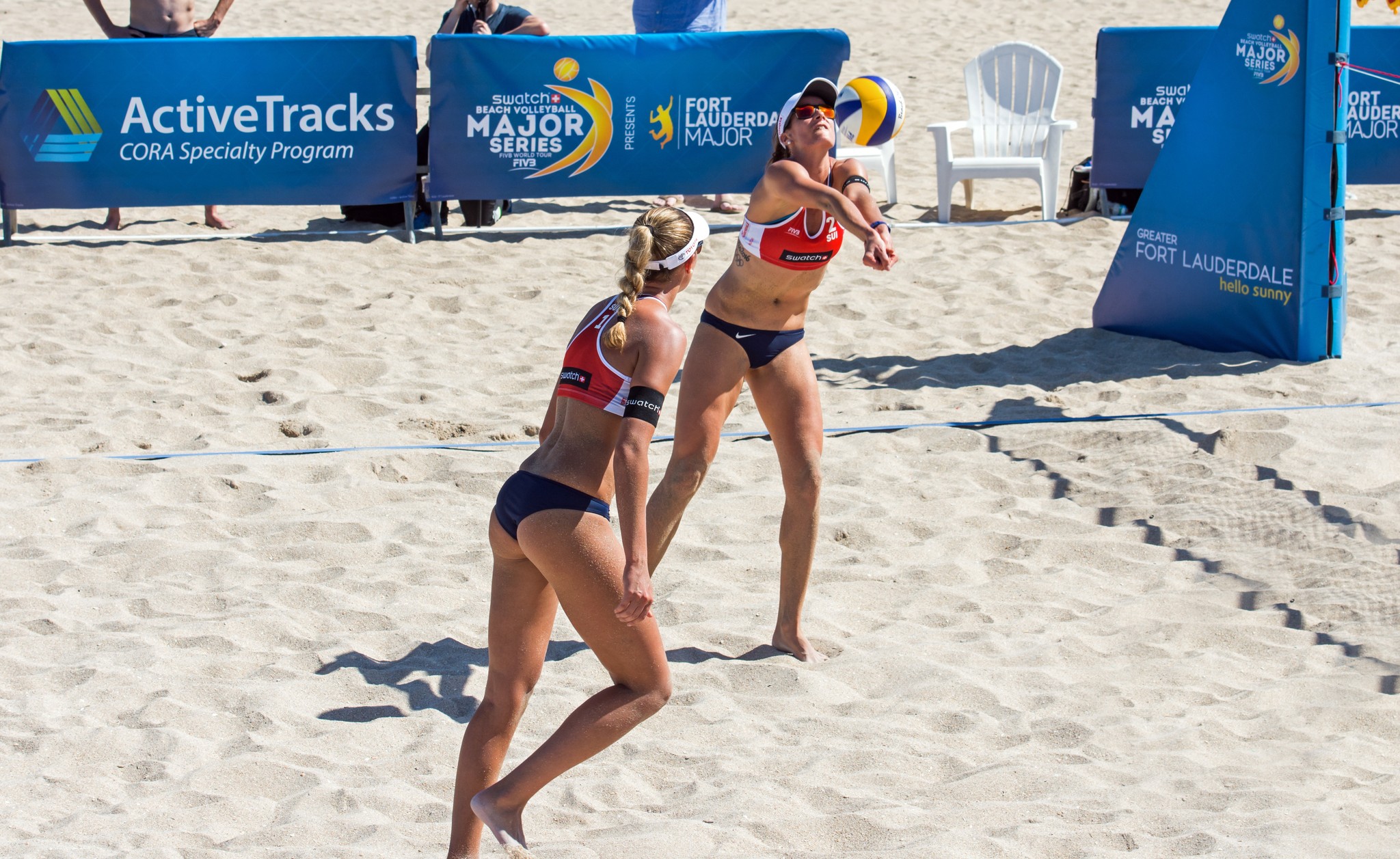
[402,213,448,231]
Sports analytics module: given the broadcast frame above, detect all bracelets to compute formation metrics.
[870,221,891,233]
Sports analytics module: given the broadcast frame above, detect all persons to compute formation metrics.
[84,0,235,230]
[447,205,710,858]
[417,0,550,224]
[646,78,898,664]
[633,0,743,215]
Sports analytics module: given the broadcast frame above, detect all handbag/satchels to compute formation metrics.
[1068,155,1145,215]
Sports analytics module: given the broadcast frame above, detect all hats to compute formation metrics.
[778,77,840,149]
[643,207,710,271]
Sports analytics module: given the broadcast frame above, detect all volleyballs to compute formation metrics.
[835,76,907,146]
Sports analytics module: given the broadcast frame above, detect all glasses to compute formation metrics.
[784,104,835,133]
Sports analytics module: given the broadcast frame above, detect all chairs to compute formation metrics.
[835,122,899,207]
[925,39,1080,226]
